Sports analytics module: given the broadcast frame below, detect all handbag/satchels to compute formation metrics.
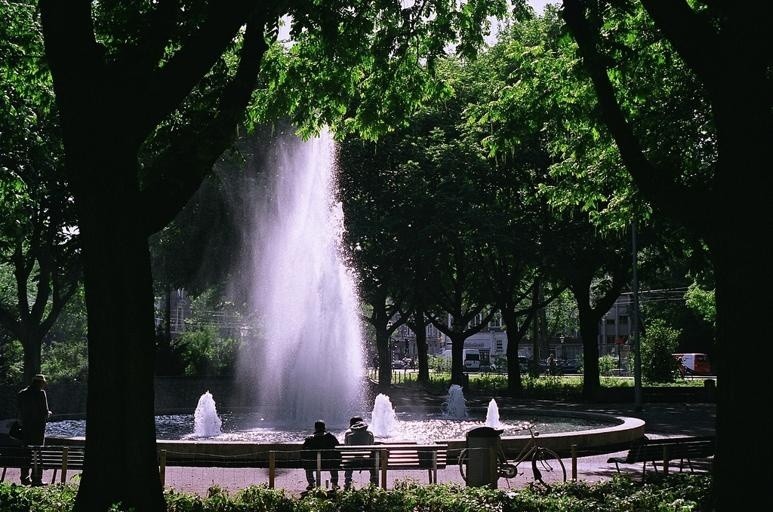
[9,421,24,442]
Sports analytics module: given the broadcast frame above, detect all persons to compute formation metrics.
[373,354,378,374]
[301,419,341,490]
[344,415,377,489]
[547,353,555,376]
[17,374,52,487]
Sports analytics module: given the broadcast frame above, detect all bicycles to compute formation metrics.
[457,417,568,488]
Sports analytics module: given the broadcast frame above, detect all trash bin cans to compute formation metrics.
[704,379,716,404]
[465,427,503,489]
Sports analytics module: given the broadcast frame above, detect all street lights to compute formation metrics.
[560,335,565,376]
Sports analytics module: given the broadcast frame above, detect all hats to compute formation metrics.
[33,374,46,382]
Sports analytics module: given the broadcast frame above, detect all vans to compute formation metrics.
[672,352,710,376]
[436,349,482,372]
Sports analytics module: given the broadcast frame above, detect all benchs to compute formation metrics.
[606,430,711,482]
[4,443,167,489]
[267,443,448,491]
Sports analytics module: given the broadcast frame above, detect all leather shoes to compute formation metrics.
[21,480,48,487]
